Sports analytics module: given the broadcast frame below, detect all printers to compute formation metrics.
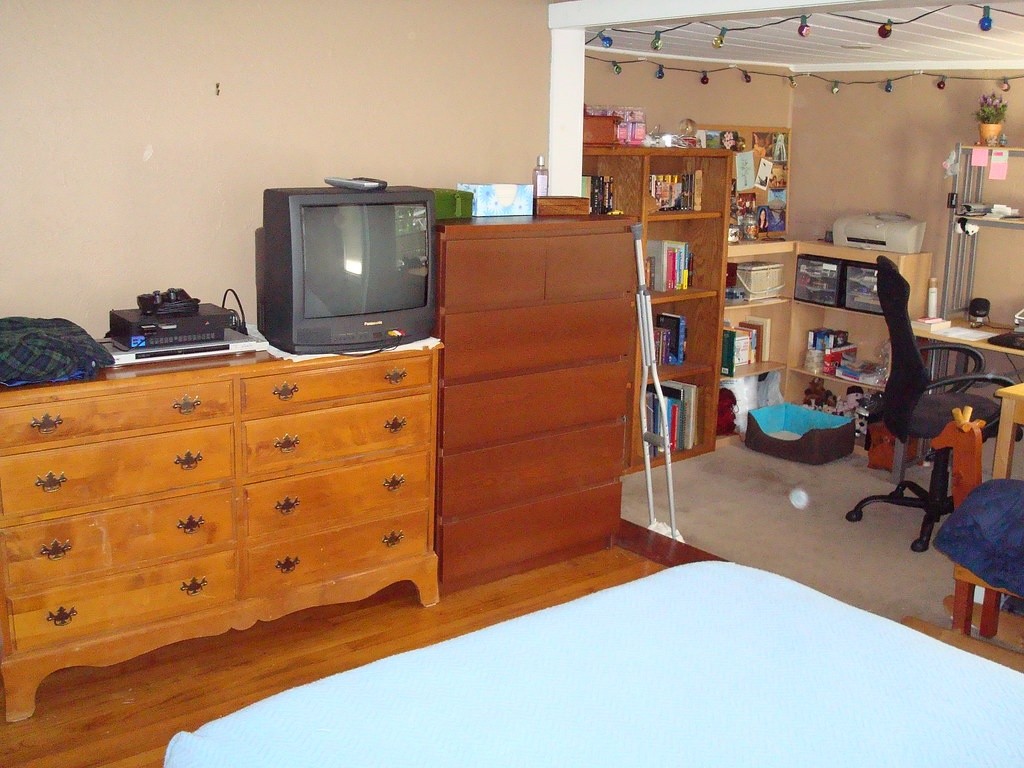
[832,210,926,254]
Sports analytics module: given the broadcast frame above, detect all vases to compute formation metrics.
[978,123,1004,145]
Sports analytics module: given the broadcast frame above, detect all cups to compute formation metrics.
[729,223,740,245]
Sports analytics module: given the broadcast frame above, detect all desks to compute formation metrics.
[162,561,1024,768]
[891,316,1024,486]
[992,383,1024,480]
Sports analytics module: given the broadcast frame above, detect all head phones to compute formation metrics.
[136,287,201,314]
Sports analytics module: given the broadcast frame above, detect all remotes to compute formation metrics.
[323,177,388,191]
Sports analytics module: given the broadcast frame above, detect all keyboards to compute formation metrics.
[987,331,1024,350]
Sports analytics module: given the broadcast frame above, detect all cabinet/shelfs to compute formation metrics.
[1,340,446,724]
[717,237,933,447]
[583,146,733,476]
[428,215,636,595]
[940,142,1023,321]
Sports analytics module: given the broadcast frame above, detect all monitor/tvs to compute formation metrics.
[254,185,435,356]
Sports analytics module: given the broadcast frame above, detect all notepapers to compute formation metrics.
[918,317,942,323]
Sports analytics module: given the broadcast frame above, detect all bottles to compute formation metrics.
[741,213,760,241]
[532,157,549,196]
[928,277,938,319]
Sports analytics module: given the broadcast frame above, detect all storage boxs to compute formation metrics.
[583,115,615,145]
[429,188,473,220]
[534,196,590,216]
[456,183,533,217]
[824,344,857,374]
[744,402,857,466]
[793,253,847,308]
[845,261,884,316]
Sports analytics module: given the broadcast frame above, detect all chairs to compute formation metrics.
[845,255,1024,553]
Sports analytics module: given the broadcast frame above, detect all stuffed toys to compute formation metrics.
[802,377,869,438]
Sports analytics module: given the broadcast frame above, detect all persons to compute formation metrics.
[758,208,768,228]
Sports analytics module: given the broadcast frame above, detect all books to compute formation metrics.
[582,175,613,214]
[649,170,702,212]
[646,240,693,292]
[720,315,771,378]
[654,312,687,366]
[646,380,699,458]
[911,317,951,332]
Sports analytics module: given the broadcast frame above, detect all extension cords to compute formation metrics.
[246,334,269,351]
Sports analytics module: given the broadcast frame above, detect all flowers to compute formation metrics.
[971,90,1007,124]
